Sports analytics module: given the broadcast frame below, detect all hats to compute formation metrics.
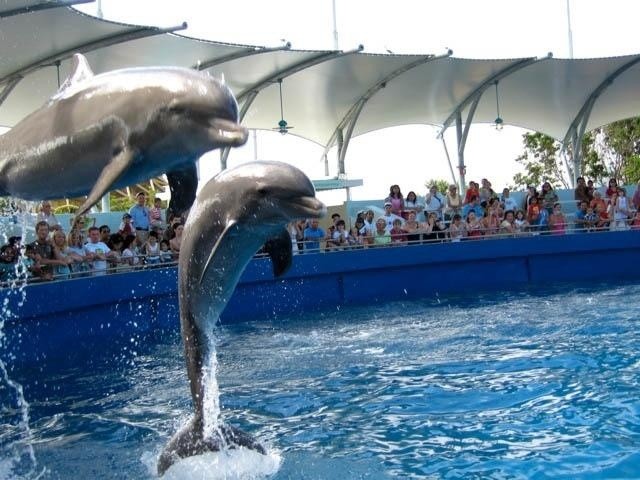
[383,203,392,208]
[122,213,132,219]
[136,192,144,198]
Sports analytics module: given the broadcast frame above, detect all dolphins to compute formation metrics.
[0,51,250,243]
[155,159,329,477]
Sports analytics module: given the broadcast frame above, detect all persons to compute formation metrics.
[0,190,187,288]
